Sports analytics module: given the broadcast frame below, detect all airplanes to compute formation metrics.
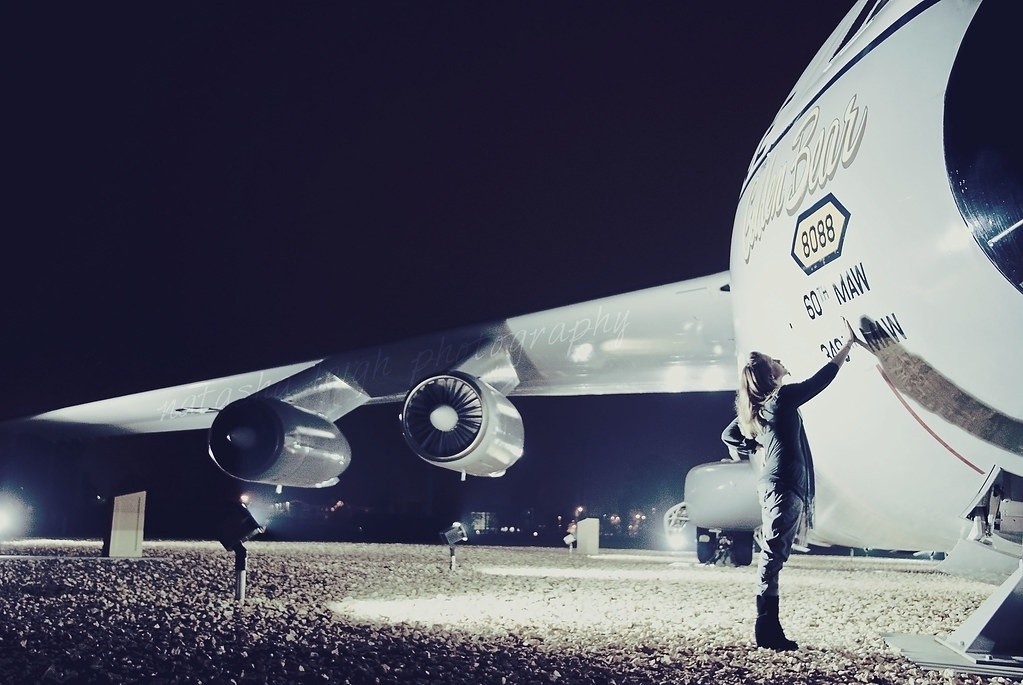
[0,0,1023,680]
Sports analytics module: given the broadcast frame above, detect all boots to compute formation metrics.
[755,595,798,650]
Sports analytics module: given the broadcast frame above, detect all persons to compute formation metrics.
[721,316,855,653]
[713,536,733,565]
